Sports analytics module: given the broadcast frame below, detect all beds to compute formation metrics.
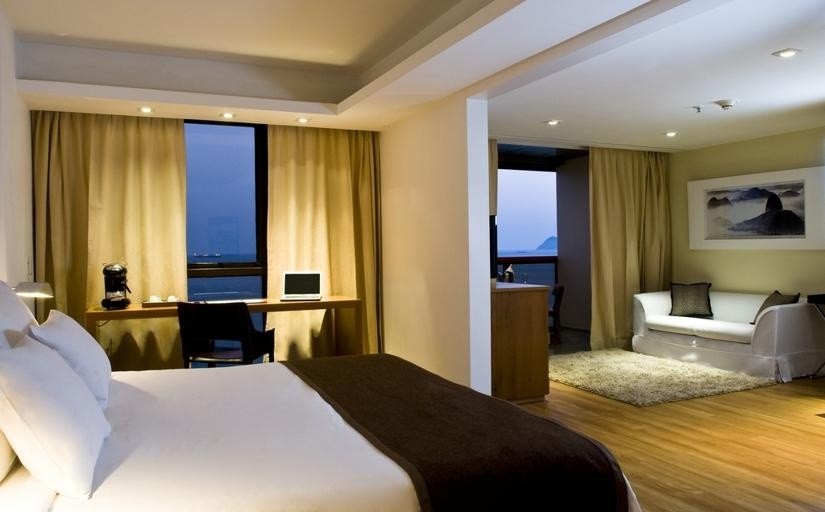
[0,280,644,510]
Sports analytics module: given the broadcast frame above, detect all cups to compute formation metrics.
[167,296,176,302]
[149,295,160,302]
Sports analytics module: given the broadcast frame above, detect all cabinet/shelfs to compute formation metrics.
[492,278,552,406]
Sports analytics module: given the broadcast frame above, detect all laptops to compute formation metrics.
[280,272,321,301]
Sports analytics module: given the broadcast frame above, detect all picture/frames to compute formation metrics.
[686,165,824,251]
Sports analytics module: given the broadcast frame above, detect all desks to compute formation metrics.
[85,293,362,370]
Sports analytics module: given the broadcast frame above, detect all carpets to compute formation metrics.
[549,346,777,406]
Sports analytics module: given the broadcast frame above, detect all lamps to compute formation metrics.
[15,281,54,320]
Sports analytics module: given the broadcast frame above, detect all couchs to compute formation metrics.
[632,289,823,383]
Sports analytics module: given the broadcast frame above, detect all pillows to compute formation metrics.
[670,282,714,318]
[750,289,800,325]
[30,309,112,410]
[0,279,43,479]
[0,335,114,501]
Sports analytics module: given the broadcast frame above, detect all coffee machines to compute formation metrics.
[100,263,131,309]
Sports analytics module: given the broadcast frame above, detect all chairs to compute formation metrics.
[177,301,274,368]
[805,293,825,379]
[549,283,565,344]
